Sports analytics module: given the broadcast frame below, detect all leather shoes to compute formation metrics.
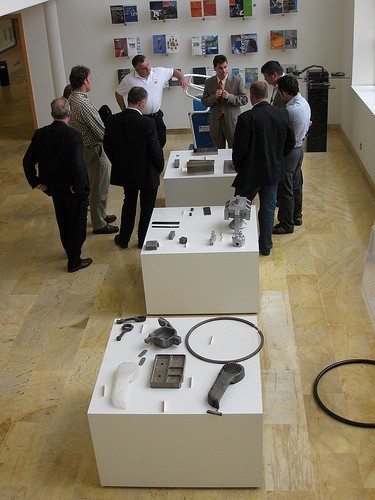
[68,258,93,273]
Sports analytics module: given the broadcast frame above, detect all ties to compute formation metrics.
[216,80,224,119]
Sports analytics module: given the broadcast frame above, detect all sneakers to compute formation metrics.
[272,224,288,234]
[93,224,119,234]
[294,217,302,225]
[104,215,117,223]
[114,235,128,248]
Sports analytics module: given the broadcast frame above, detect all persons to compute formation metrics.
[23,97,93,273]
[115,55,188,149]
[201,54,248,149]
[103,86,165,248]
[261,60,287,108]
[67,66,119,234]
[272,75,312,235]
[231,81,296,256]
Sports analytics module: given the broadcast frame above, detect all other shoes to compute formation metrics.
[259,246,270,256]
